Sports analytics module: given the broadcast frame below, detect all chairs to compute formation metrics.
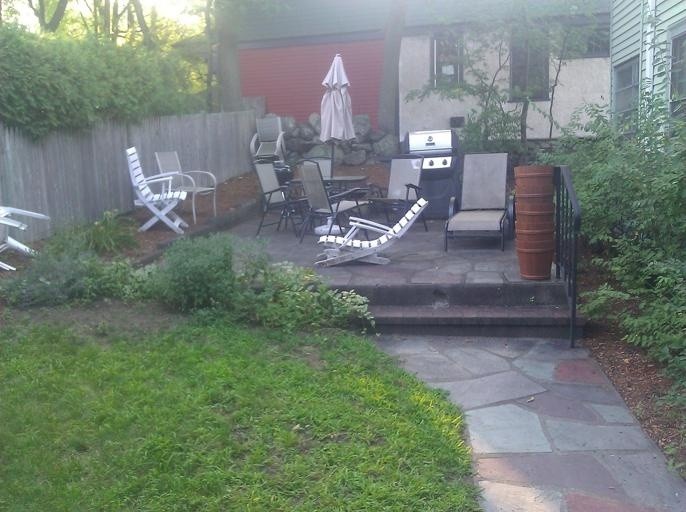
[249,115,431,269]
[125,145,218,235]
[443,153,514,253]
[0,205,49,274]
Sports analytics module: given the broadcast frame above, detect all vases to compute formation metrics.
[513,164,559,279]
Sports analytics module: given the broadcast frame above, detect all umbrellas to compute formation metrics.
[317,54,358,177]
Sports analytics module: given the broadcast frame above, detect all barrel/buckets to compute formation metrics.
[513,164,556,281]
